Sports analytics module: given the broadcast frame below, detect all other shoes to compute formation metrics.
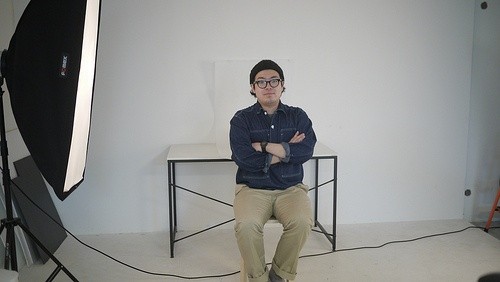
[269,269,290,282]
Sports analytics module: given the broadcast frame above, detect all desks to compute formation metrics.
[166,143,338,259]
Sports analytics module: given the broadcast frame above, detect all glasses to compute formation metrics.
[252,79,283,89]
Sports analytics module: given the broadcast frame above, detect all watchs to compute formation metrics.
[260,141,268,152]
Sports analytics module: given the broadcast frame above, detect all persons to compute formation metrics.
[229,60,317,282]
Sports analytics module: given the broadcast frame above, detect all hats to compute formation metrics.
[250,59,285,82]
